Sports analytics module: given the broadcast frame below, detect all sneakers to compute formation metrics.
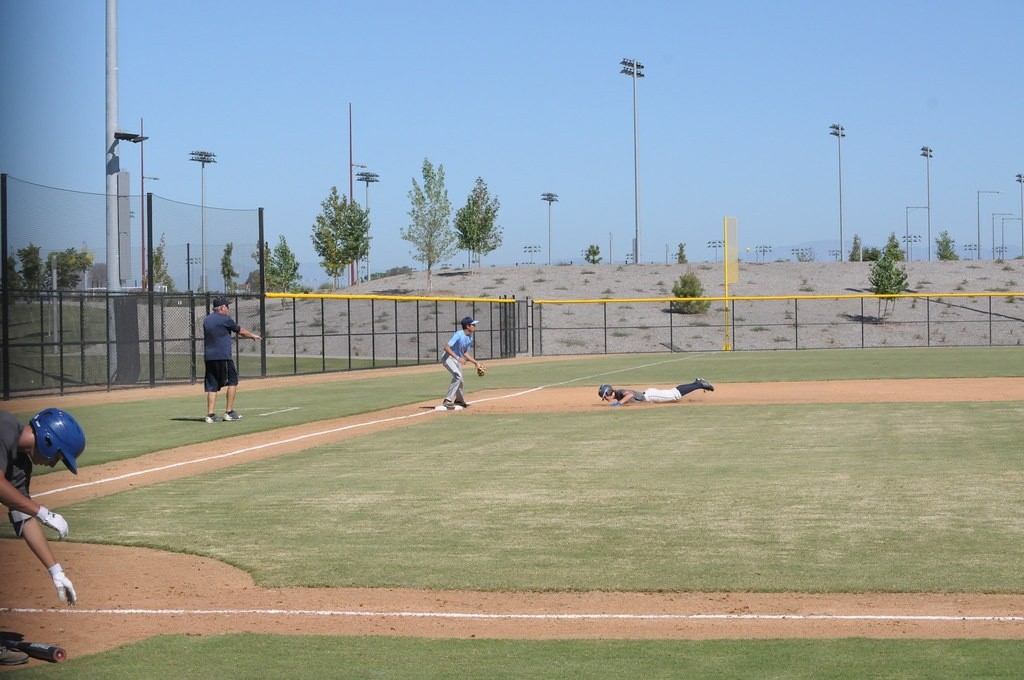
[0,646,29,665]
[695,378,714,393]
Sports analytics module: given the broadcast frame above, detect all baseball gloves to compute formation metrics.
[475,362,486,376]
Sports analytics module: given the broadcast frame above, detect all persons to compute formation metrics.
[203,297,263,424]
[0,408,85,665]
[441,317,486,409]
[598,378,714,408]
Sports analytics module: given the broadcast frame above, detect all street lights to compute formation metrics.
[187,151,216,291]
[919,146,936,262]
[992,213,1015,261]
[1015,171,1024,259]
[830,125,847,260]
[976,190,1003,260]
[620,58,645,263]
[542,193,559,264]
[356,172,379,282]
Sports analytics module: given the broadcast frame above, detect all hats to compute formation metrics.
[461,317,478,326]
[213,297,232,307]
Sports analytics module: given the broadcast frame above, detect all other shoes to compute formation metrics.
[454,399,471,408]
[223,409,242,421]
[206,416,223,424]
[443,398,455,408]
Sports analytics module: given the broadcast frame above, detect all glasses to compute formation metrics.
[470,324,475,327]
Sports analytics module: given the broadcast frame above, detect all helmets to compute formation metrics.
[30,408,85,475]
[598,385,612,401]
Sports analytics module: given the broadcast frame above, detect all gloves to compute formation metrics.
[48,563,77,606]
[36,505,68,539]
[609,401,621,408]
